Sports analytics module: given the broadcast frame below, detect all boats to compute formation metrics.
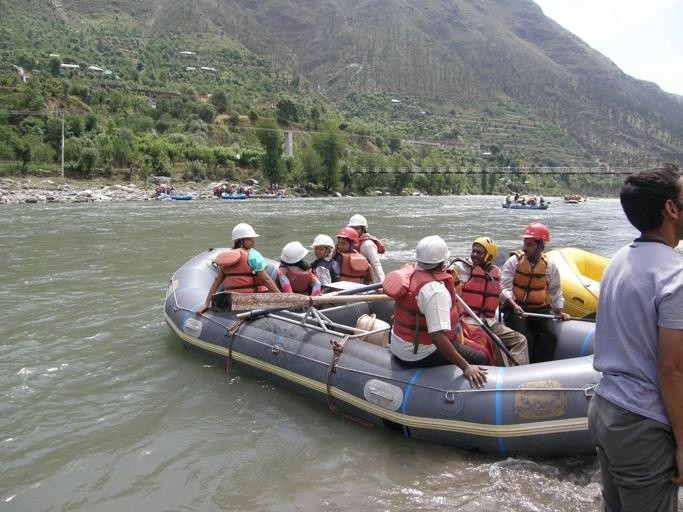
[160,192,193,200]
[221,193,246,199]
[163,247,603,460]
[562,200,577,203]
[537,245,611,322]
[502,201,548,209]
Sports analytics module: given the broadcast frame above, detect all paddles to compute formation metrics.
[230,292,396,312]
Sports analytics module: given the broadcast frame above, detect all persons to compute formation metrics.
[587,165,681,510]
[458,321,496,366]
[196,223,279,314]
[271,241,320,296]
[270,190,287,196]
[387,235,488,388]
[505,194,544,205]
[347,214,385,283]
[499,221,570,362]
[564,193,581,202]
[213,185,250,198]
[155,185,174,195]
[308,234,340,293]
[449,237,529,366]
[331,226,383,293]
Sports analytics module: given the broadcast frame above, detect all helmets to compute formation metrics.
[231,222,260,240]
[518,222,550,241]
[414,235,450,264]
[281,214,367,264]
[472,237,498,263]
